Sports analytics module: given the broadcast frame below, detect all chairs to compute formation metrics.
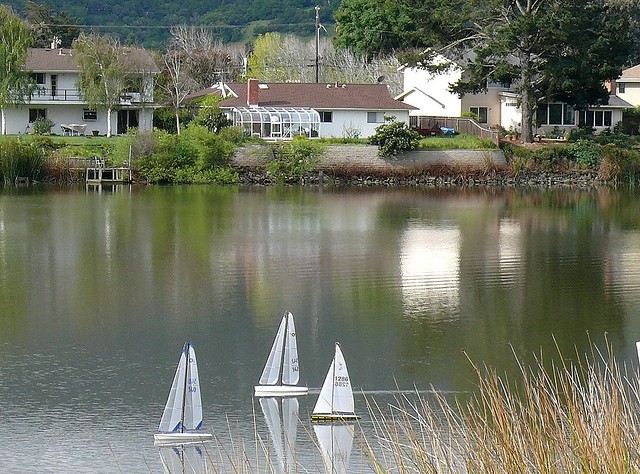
[61,125,70,136]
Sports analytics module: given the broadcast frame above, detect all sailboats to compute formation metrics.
[151,341,213,441]
[253,310,310,392]
[311,341,361,421]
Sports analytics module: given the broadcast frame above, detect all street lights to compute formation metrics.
[315,5,321,83]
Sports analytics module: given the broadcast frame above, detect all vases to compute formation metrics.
[92,131,99,136]
